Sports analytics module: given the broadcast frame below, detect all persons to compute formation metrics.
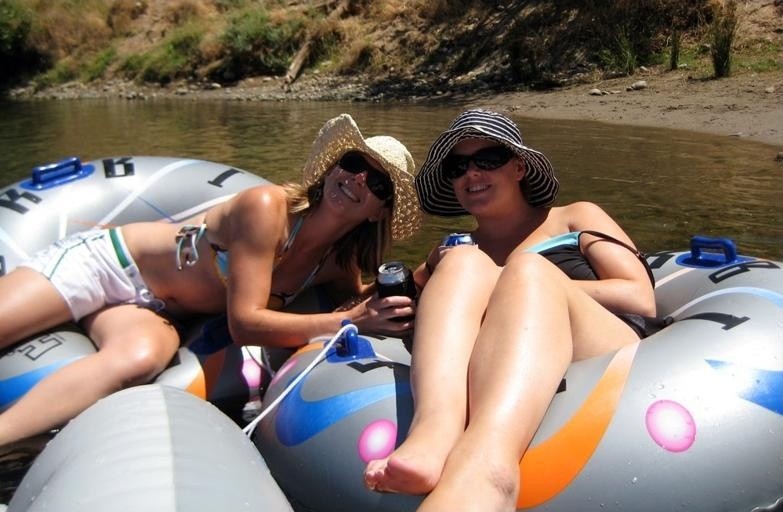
[0,112,423,446]
[363,108,658,512]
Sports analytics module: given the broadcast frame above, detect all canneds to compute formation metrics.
[376,262,418,322]
[444,231,472,245]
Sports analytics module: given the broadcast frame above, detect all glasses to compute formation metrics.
[442,146,513,179]
[339,151,392,200]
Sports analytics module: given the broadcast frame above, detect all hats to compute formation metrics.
[415,109,559,216]
[303,113,423,240]
